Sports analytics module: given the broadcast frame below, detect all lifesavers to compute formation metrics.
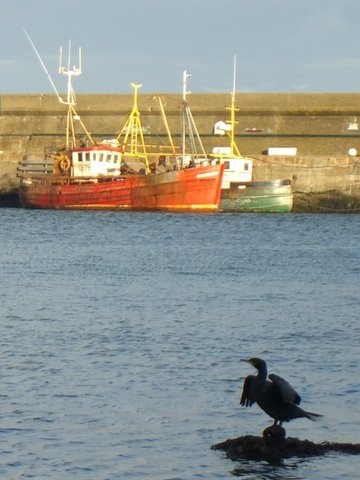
[60,159,70,170]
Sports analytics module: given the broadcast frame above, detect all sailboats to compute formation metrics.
[175,54,293,213]
[16,28,227,212]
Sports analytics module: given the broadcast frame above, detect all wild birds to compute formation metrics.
[239,357,326,430]
